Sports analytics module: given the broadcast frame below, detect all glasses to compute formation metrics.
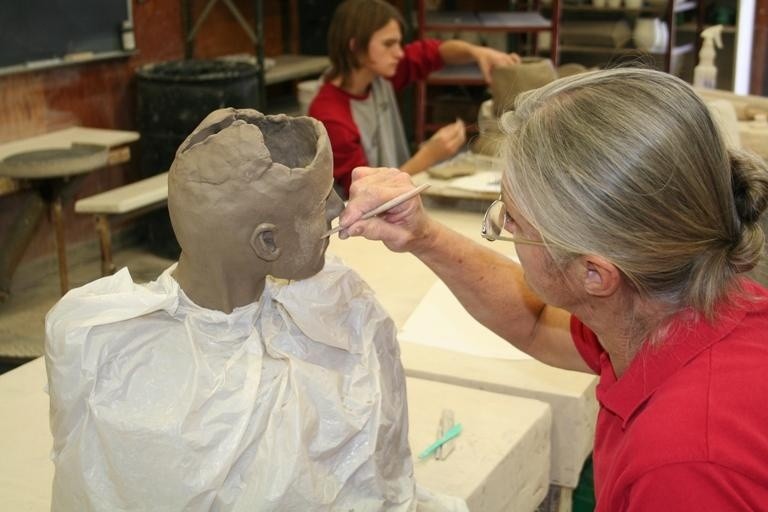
[481,194,561,248]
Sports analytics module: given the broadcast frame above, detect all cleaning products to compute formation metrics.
[693,24,724,88]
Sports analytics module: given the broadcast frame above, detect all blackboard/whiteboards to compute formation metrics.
[1,0,142,78]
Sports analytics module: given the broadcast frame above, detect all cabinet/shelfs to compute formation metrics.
[418,0,701,144]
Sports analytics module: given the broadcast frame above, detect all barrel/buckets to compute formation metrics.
[297,80,320,116]
[133,59,264,257]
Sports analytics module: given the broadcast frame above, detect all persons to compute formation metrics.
[307,0,521,201]
[167,107,348,315]
[339,67,768,512]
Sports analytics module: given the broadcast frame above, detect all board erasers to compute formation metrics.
[64,51,93,62]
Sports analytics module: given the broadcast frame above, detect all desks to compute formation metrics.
[2,126,601,512]
